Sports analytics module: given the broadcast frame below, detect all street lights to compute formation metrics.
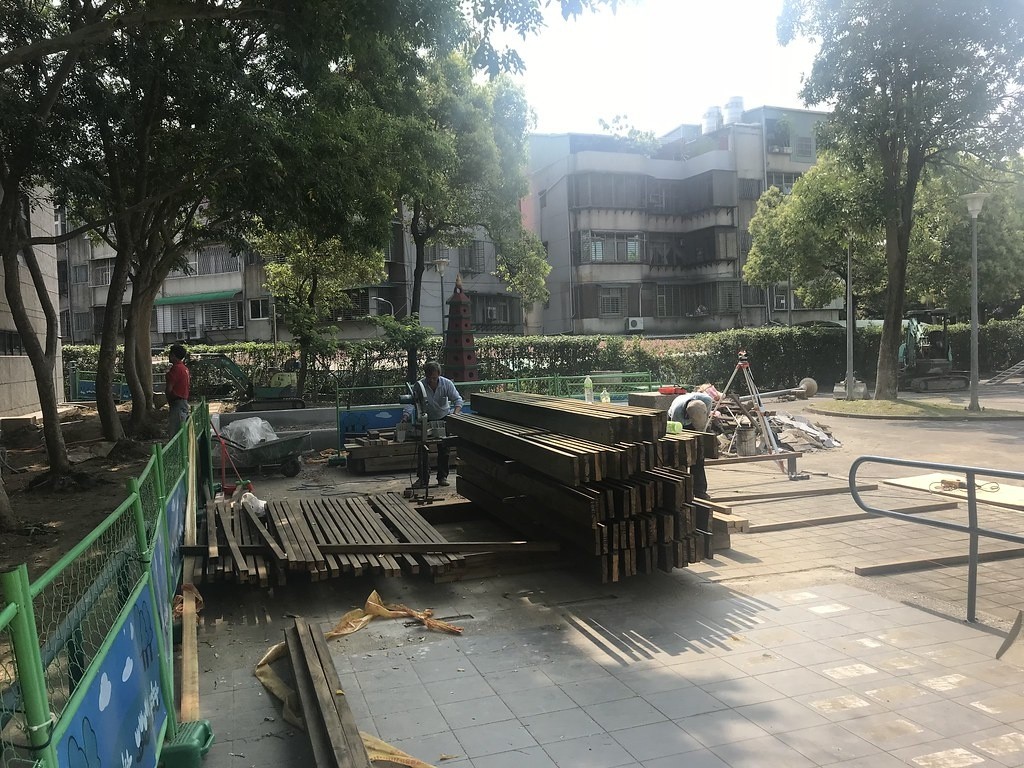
[960,192,991,415]
[840,220,856,402]
[434,257,452,346]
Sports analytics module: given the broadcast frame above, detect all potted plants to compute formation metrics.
[770,112,793,153]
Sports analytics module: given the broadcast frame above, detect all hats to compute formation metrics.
[687,399,707,428]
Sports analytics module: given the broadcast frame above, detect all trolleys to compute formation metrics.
[212,430,316,478]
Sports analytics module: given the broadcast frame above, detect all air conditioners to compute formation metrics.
[486,307,497,320]
[626,318,643,330]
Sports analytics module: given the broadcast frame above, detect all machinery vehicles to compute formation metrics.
[212,353,306,414]
[899,310,976,394]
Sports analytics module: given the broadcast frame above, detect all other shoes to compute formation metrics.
[438,478,449,486]
[695,493,710,499]
[412,479,429,488]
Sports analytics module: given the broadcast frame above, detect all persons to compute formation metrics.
[667,393,713,501]
[165,346,190,440]
[398,362,462,487]
